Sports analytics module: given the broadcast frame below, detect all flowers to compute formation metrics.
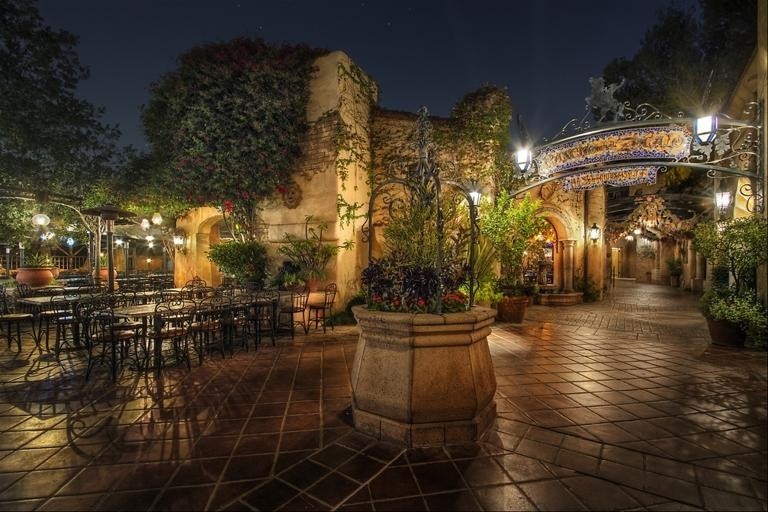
[360,251,470,313]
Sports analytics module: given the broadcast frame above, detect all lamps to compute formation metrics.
[139,206,163,231]
[172,227,189,257]
[693,113,719,146]
[515,146,534,174]
[588,222,600,245]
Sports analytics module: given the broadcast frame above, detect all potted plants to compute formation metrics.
[92,255,118,281]
[665,257,684,287]
[476,186,551,323]
[16,252,54,287]
[687,214,767,350]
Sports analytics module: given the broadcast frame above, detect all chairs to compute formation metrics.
[278,285,310,340]
[17,276,281,384]
[0,285,40,352]
[308,282,337,334]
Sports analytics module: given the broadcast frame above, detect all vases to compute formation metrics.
[349,304,502,446]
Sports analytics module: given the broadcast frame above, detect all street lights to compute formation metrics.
[80,204,136,295]
